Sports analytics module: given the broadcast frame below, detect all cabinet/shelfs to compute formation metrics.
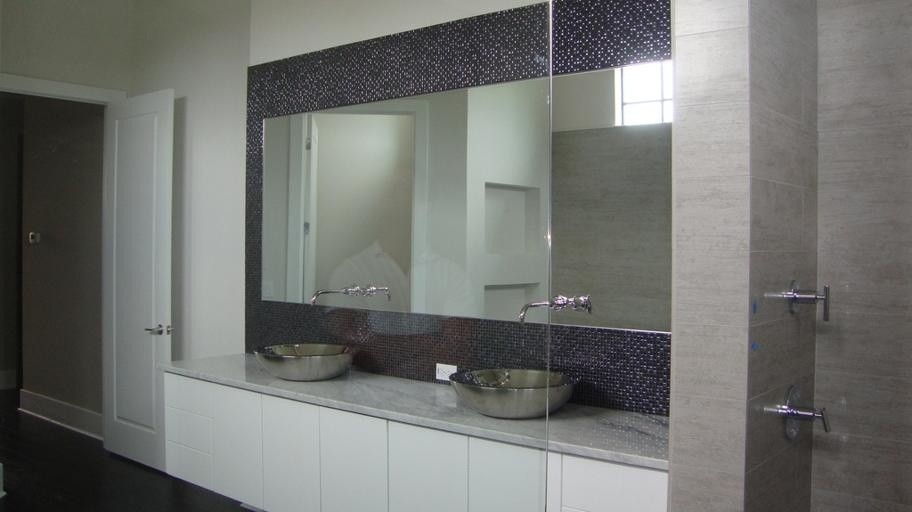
[211,385,561,511]
[163,372,213,490]
[561,456,669,512]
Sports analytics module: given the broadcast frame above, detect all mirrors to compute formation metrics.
[258,51,672,339]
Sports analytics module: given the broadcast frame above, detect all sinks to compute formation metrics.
[253,340,355,381]
[450,369,583,420]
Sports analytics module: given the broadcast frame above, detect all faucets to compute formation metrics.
[361,285,392,301]
[563,295,595,311]
[310,286,361,303]
[517,293,562,324]
